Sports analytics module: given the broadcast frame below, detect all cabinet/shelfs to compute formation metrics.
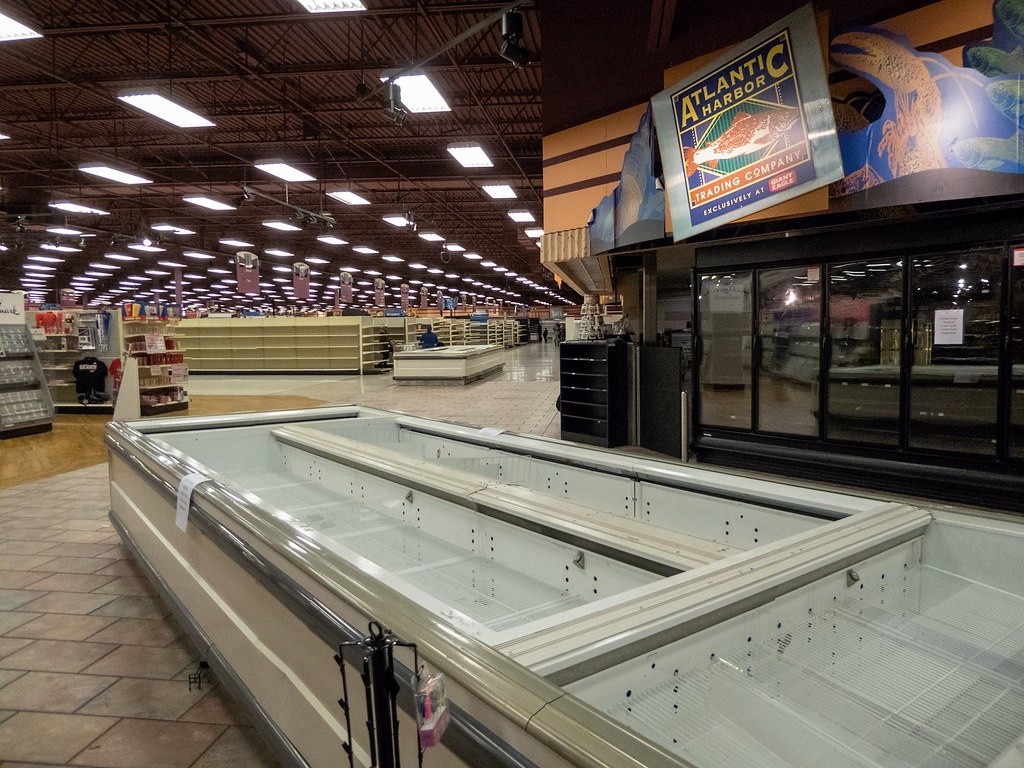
[500,320,510,346]
[176,316,389,375]
[515,322,527,345]
[558,340,629,448]
[464,320,474,344]
[0,292,56,441]
[25,308,192,417]
[702,312,750,385]
[416,318,442,349]
[492,320,501,344]
[373,316,424,367]
[440,319,459,345]
[481,320,493,343]
[509,320,517,346]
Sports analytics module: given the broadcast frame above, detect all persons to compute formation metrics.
[378,324,393,372]
[543,328,548,342]
[537,322,542,342]
[420,326,438,349]
[551,323,565,346]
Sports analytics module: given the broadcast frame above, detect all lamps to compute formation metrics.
[53,237,63,247]
[356,83,378,108]
[234,251,261,268]
[233,192,255,207]
[293,262,310,277]
[288,208,333,235]
[498,8,535,70]
[340,272,354,285]
[374,278,385,289]
[42,235,52,246]
[383,82,408,126]
